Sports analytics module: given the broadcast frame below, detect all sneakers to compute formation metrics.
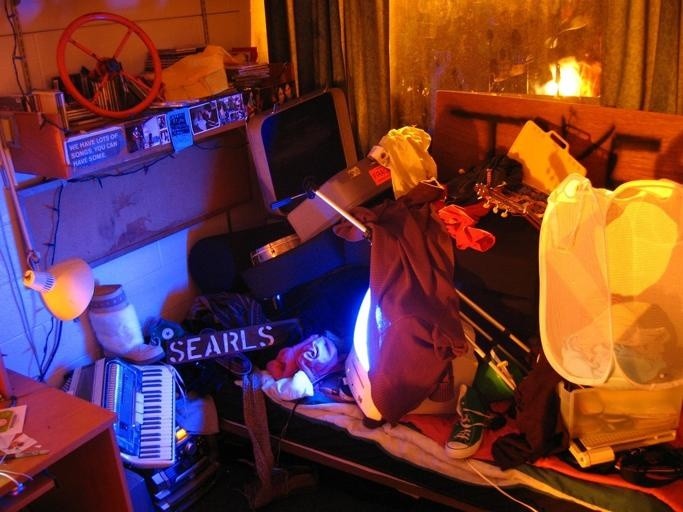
[441,389,488,459]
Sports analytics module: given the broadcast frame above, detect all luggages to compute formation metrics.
[245,86,391,243]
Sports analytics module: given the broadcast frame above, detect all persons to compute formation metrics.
[485,0,608,102]
[128,82,294,150]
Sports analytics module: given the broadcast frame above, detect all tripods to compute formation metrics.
[270,180,535,389]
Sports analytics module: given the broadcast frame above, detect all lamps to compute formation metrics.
[22,258,95,321]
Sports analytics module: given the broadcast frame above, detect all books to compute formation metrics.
[0,45,272,134]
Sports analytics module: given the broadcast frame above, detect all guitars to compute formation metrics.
[475,183,543,230]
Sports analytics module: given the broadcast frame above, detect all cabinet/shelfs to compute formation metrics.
[0,367,132,512]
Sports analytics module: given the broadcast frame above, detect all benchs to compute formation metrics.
[184,90,683,512]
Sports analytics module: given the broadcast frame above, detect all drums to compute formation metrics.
[251,232,300,266]
